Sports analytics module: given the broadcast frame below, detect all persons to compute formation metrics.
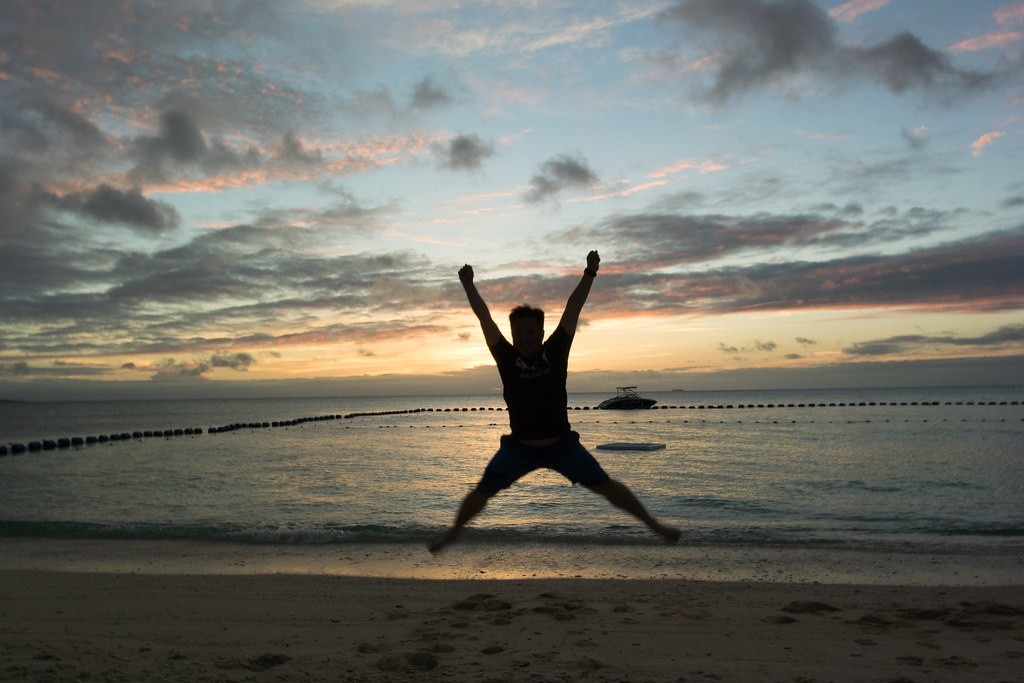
[426,247,684,558]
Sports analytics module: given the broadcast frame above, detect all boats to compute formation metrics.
[596,386,658,409]
